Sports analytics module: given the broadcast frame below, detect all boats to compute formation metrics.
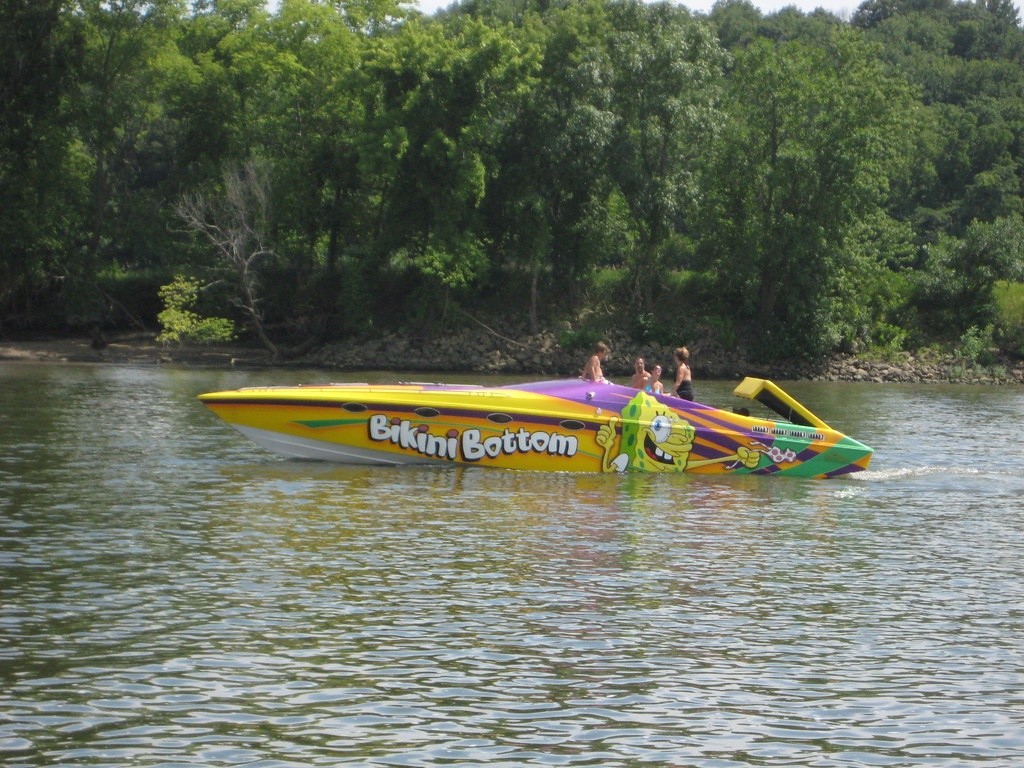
[197,377,874,479]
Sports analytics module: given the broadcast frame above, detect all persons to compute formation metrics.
[578,341,614,385]
[631,364,663,395]
[663,347,694,401]
[631,356,651,387]
